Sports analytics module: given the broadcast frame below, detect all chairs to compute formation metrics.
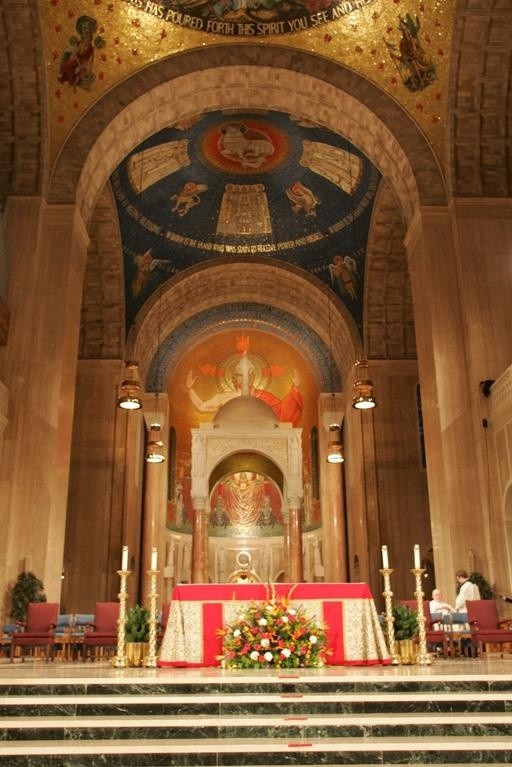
[399,599,512,659]
[0,603,118,663]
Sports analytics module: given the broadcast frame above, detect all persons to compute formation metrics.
[59,29,97,95]
[453,568,480,614]
[183,355,305,427]
[327,252,361,302]
[129,245,174,300]
[428,588,454,621]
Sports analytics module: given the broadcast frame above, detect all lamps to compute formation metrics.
[326,144,377,463]
[118,145,166,464]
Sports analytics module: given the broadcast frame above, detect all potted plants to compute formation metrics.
[126,604,159,666]
[386,604,420,665]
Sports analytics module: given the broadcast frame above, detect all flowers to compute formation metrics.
[218,596,335,669]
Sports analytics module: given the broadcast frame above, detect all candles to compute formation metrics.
[413,544,421,568]
[151,548,157,570]
[122,546,128,570]
[382,545,389,569]
